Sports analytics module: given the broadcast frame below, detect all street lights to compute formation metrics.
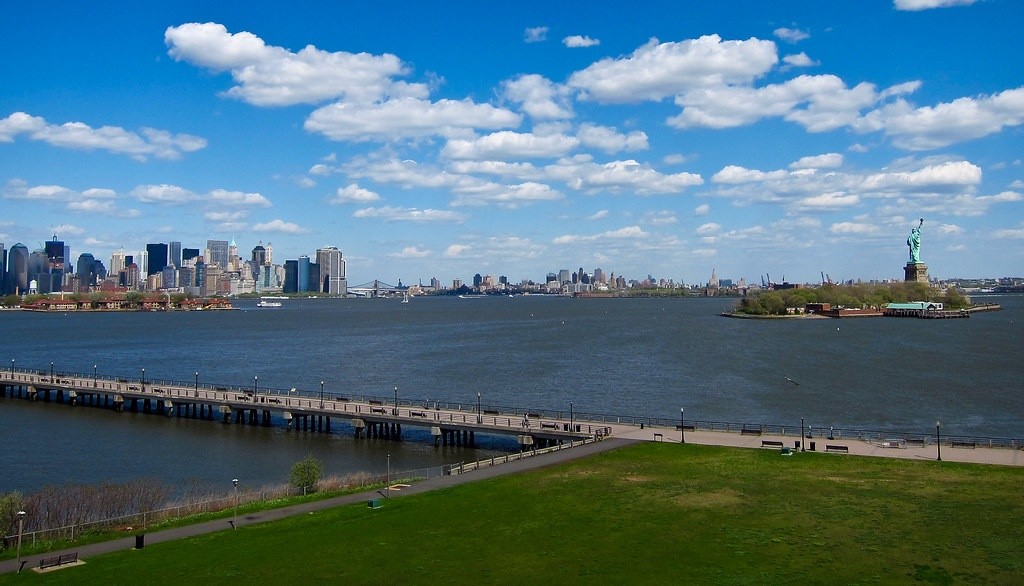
[255,376,257,402]
[51,362,54,383]
[477,392,481,423]
[321,381,324,409]
[570,401,573,431]
[17,511,26,573]
[142,368,144,392]
[801,417,806,451]
[196,372,198,393]
[680,407,685,443]
[12,359,14,378]
[395,387,397,415]
[232,479,239,530]
[94,365,96,387]
[936,420,941,460]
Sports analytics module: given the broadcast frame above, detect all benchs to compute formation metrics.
[906,438,925,448]
[524,413,541,419]
[952,442,975,449]
[542,423,560,430]
[373,408,387,414]
[237,396,255,401]
[269,399,282,404]
[760,441,783,448]
[825,445,852,453]
[484,411,499,416]
[369,400,383,406]
[741,429,762,436]
[411,412,427,418]
[336,397,351,403]
[676,425,701,431]
[40,552,78,570]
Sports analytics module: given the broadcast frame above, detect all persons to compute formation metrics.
[907,216,924,262]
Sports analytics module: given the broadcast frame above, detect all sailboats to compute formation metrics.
[401,293,409,304]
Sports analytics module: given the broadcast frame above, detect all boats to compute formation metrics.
[257,301,282,308]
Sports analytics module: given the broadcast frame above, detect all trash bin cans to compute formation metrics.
[391,407,399,416]
[794,440,800,450]
[641,422,643,429]
[564,423,568,431]
[56,379,60,384]
[576,425,580,432]
[810,441,816,451]
[261,396,265,403]
